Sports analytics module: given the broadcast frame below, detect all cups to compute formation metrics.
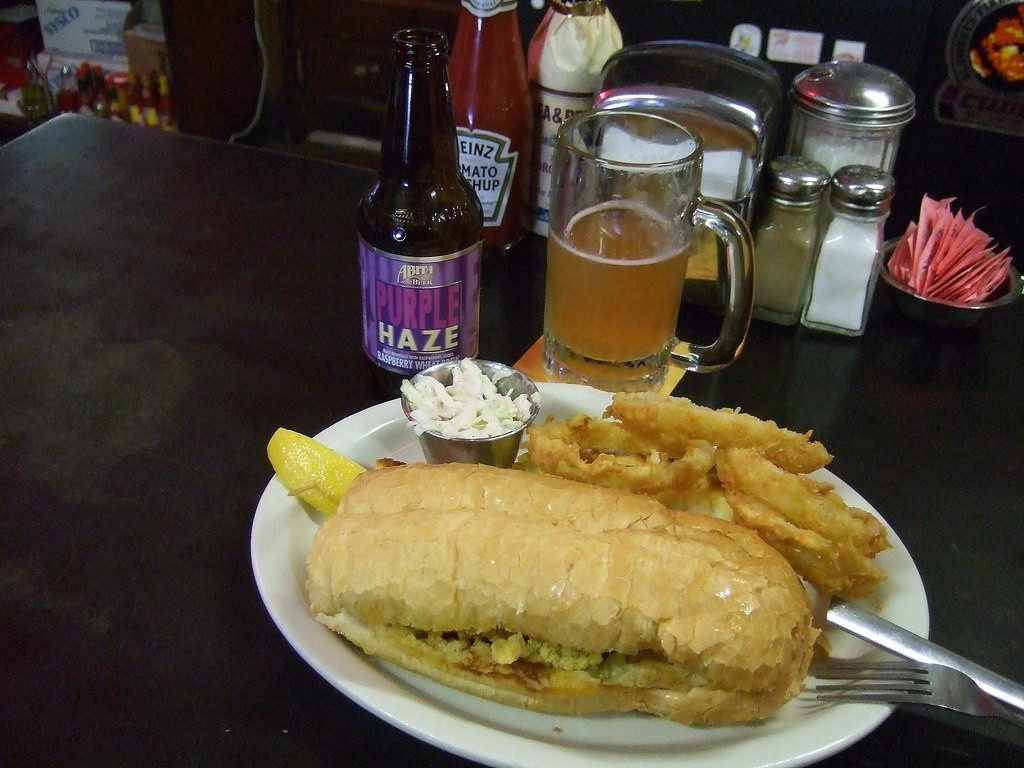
[543,109,755,392]
[401,360,539,467]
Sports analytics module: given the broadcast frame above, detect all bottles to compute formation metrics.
[783,60,916,175]
[355,26,483,387]
[23,53,180,133]
[755,155,831,327]
[448,0,533,251]
[522,0,623,240]
[800,164,896,336]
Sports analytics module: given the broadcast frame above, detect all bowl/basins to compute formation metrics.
[881,235,1023,329]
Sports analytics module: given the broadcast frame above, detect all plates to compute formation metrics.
[247,382,929,767]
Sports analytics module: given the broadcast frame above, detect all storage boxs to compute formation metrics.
[0,0,146,106]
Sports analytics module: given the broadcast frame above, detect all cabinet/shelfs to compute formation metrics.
[0,1,1024,214]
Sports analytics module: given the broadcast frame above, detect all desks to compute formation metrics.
[0,114,1024,768]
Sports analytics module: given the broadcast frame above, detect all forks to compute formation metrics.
[812,660,1024,728]
